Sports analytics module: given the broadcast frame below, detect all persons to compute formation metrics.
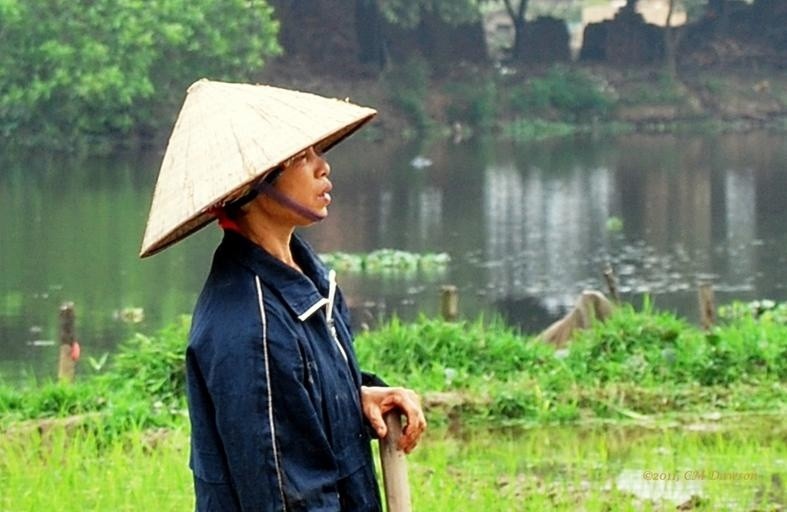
[137,77,428,512]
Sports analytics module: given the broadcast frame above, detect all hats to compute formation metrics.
[138,76,378,260]
